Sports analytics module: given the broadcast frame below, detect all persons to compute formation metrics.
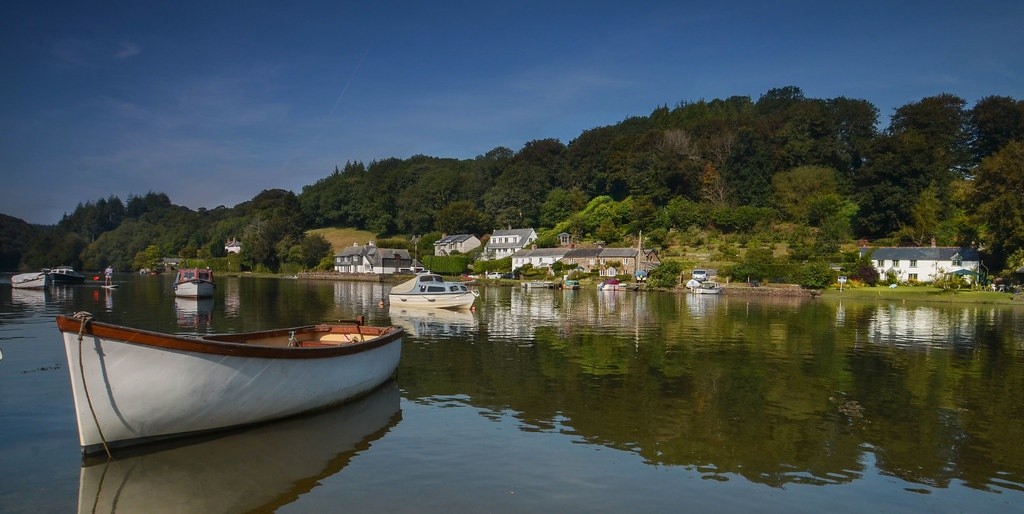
[103,265,114,286]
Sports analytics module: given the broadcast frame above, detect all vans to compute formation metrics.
[690,269,709,282]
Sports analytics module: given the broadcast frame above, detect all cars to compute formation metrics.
[500,272,519,280]
[486,272,504,280]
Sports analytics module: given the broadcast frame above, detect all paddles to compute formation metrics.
[93,272,108,280]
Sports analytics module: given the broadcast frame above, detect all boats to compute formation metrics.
[150,270,158,276]
[56,311,403,457]
[175,296,215,330]
[597,277,627,292]
[685,279,701,289]
[11,268,51,290]
[389,273,481,308]
[389,306,474,328]
[690,281,724,294]
[173,267,216,298]
[47,265,85,285]
[562,280,581,290]
[77,369,405,514]
[11,288,52,305]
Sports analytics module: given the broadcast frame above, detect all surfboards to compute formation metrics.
[101,285,118,287]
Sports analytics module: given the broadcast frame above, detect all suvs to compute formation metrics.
[414,269,434,275]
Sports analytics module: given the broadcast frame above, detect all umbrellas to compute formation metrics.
[946,269,979,280]
